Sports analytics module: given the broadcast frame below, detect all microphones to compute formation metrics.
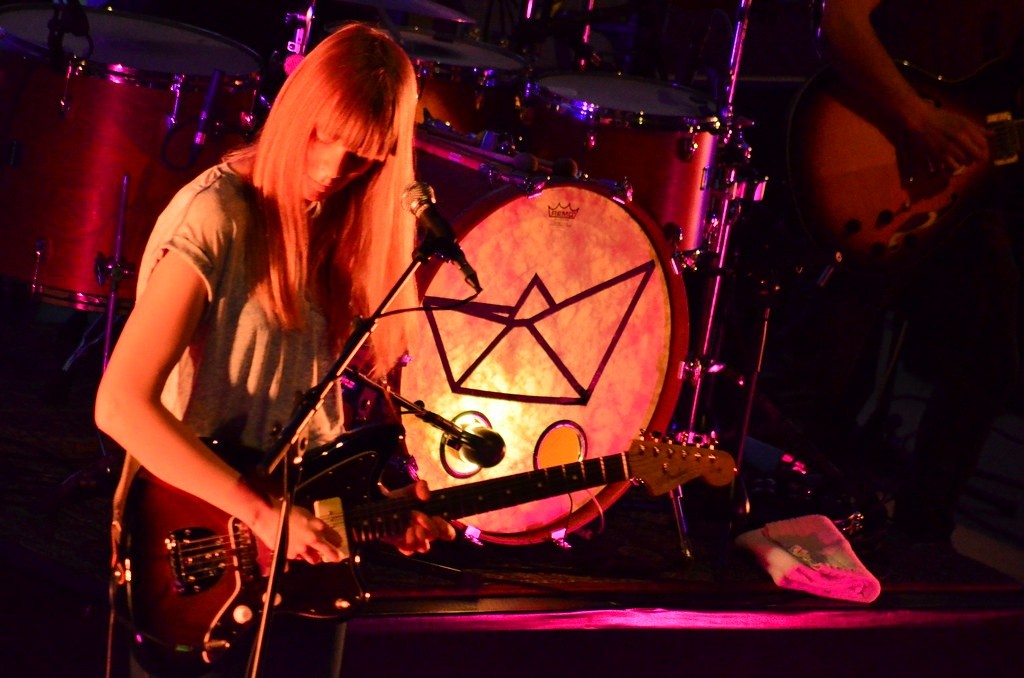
[401,181,479,288]
[459,422,508,467]
[189,67,225,164]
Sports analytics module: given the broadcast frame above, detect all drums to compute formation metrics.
[369,1,481,41]
[343,131,698,551]
[2,1,267,324]
[519,68,722,273]
[391,24,535,146]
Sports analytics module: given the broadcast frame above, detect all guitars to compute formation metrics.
[783,49,1024,285]
[112,407,741,677]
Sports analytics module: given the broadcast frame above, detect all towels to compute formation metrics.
[734,514,882,607]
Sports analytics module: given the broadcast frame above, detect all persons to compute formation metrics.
[813,0,1024,176]
[95,25,456,563]
